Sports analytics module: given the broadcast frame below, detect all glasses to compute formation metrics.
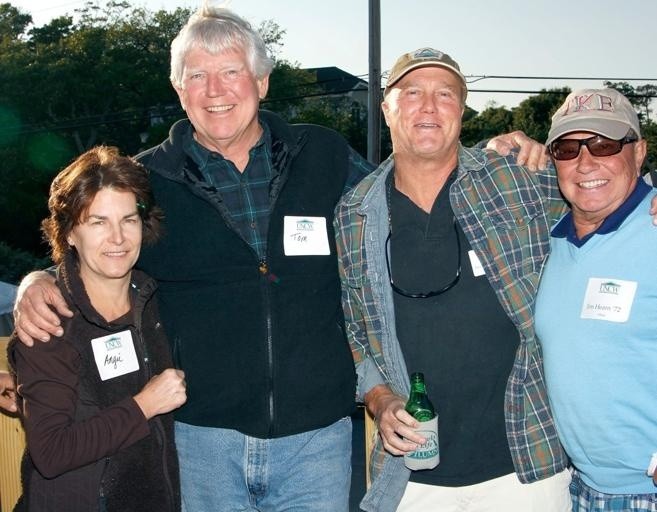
[381,221,466,300]
[548,134,639,162]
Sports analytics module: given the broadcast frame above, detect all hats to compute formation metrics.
[542,87,645,146]
[385,46,468,94]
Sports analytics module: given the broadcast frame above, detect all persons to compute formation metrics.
[11,2,549,512]
[1,366,20,416]
[334,47,657,512]
[532,87,656,512]
[5,144,189,512]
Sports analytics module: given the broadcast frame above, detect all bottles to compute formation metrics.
[404,372,441,471]
